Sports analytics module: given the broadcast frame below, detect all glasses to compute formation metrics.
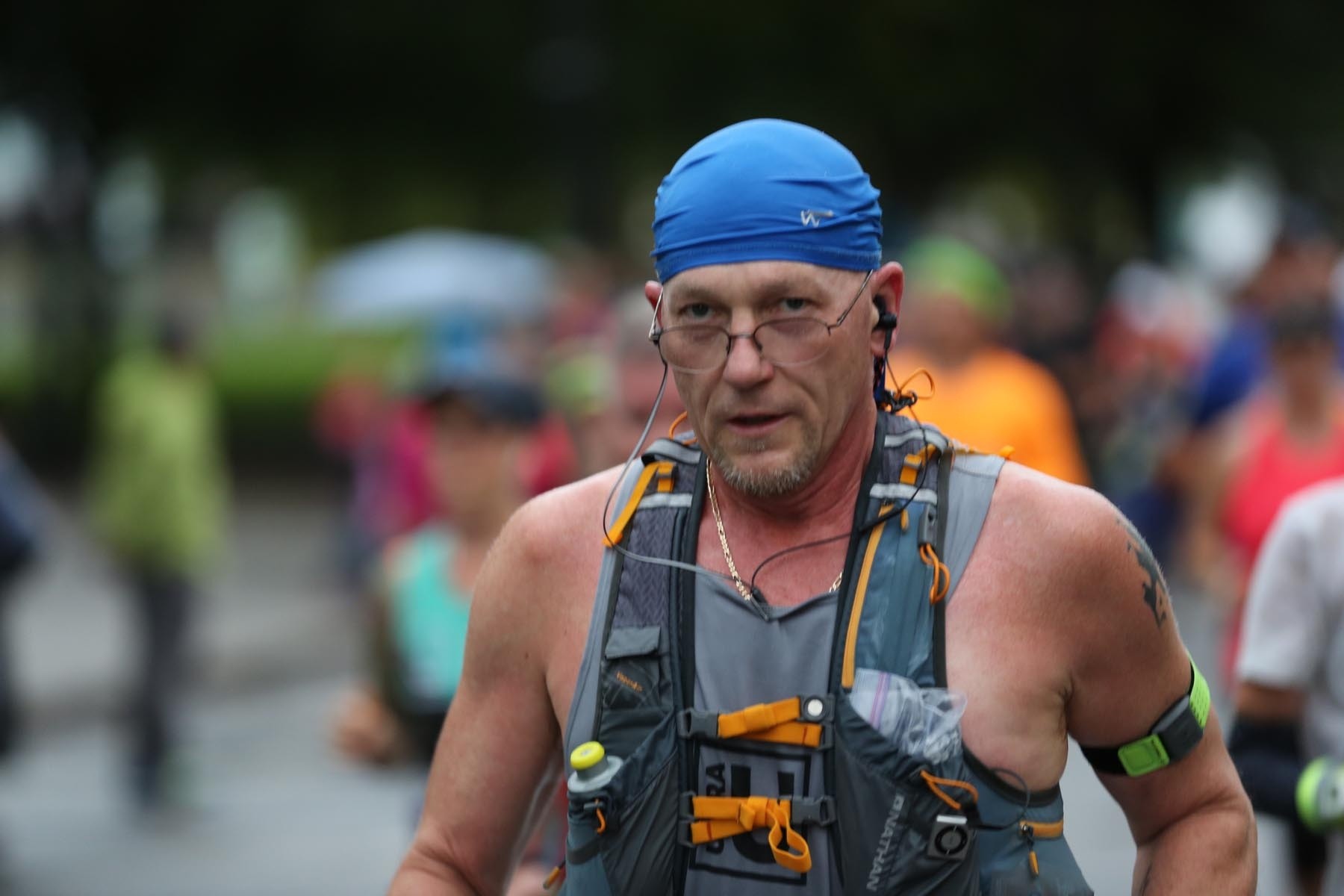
[647,269,878,374]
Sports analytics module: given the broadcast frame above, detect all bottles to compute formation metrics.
[567,740,625,795]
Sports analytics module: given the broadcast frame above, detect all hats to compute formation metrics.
[650,117,884,284]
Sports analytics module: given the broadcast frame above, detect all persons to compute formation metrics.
[378,118,1264,896]
[85,163,1343,896]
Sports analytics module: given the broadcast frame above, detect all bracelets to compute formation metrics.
[1081,655,1211,780]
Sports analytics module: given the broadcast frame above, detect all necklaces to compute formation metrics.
[705,457,844,600]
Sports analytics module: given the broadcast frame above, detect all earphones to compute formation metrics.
[871,296,897,351]
